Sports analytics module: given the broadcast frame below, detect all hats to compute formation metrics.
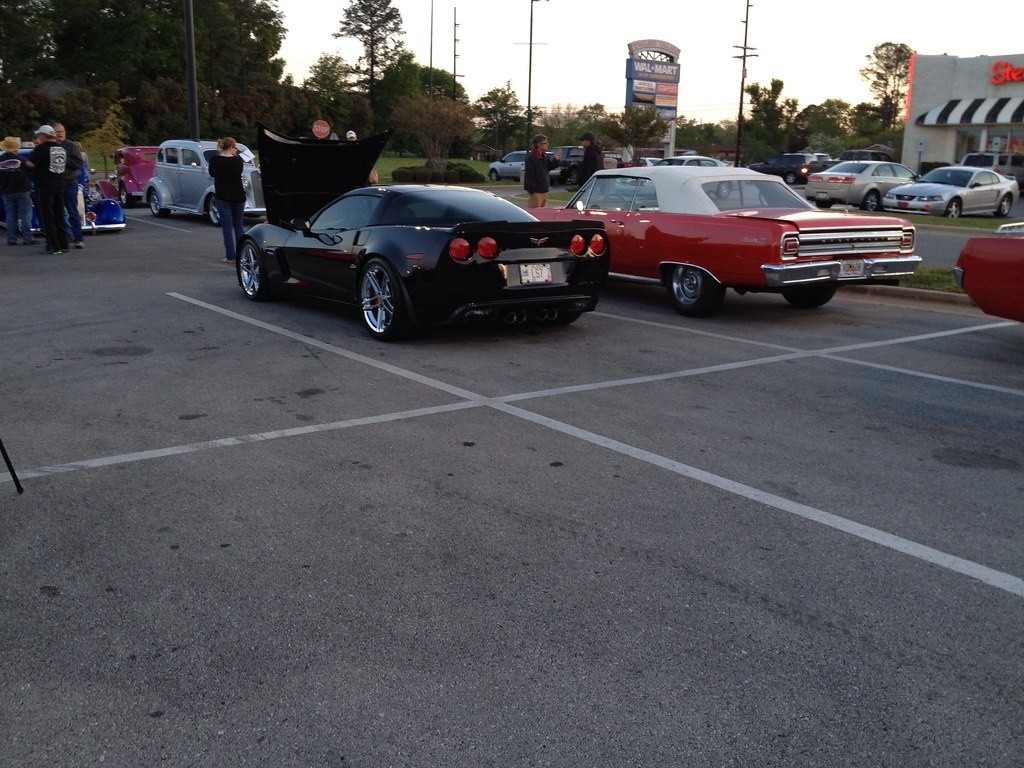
[579,133,596,141]
[346,130,356,139]
[0,136,21,151]
[34,125,56,138]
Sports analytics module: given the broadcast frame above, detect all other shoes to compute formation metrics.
[223,258,235,264]
[7,238,85,256]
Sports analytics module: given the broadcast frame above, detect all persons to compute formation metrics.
[342,131,378,192]
[69,141,93,202]
[208,137,248,265]
[577,132,607,204]
[256,215,270,228]
[29,132,59,234]
[523,132,564,212]
[0,136,41,248]
[48,121,85,250]
[24,125,74,257]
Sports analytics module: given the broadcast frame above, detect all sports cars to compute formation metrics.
[230,138,611,339]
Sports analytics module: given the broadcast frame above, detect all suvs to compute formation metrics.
[960,151,1024,186]
[747,153,817,185]
[552,145,584,166]
[487,150,561,188]
[603,151,623,168]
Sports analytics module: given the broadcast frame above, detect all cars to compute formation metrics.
[142,139,266,229]
[629,157,663,167]
[883,165,1018,219]
[521,165,922,320]
[805,159,922,211]
[614,156,733,205]
[95,146,158,209]
[0,142,127,235]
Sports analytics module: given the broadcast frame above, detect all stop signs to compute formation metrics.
[312,120,330,138]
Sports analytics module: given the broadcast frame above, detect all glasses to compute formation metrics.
[233,146,238,150]
[541,142,547,145]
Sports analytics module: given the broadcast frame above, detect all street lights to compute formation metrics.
[525,0,550,152]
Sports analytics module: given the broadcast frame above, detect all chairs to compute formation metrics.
[399,208,416,219]
[441,199,473,220]
